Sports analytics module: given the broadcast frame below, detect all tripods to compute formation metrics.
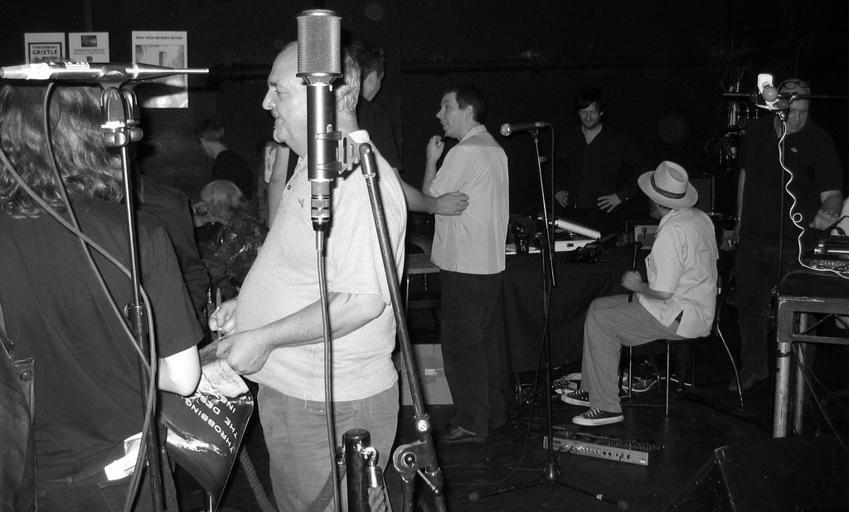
[469,235,628,508]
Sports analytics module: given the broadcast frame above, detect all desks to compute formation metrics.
[772,247,849,440]
[388,343,455,512]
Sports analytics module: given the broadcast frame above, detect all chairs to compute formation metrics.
[687,175,720,217]
[404,253,443,317]
[628,249,744,417]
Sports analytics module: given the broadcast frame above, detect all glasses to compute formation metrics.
[778,80,810,93]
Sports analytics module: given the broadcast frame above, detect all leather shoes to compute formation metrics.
[438,425,485,444]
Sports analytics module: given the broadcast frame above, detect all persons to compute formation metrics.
[540,86,643,229]
[210,40,408,511]
[420,83,515,444]
[196,119,258,201]
[560,157,717,426]
[722,79,843,403]
[346,42,470,217]
[109,124,213,317]
[195,180,269,301]
[1,77,201,510]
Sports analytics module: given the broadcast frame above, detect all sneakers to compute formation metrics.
[561,390,591,407]
[572,408,625,426]
[729,371,757,391]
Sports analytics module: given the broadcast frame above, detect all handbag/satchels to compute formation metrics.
[0,312,39,511]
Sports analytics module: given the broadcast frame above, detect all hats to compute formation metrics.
[638,161,698,209]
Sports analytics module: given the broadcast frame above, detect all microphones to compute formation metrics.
[296,9,343,252]
[499,121,544,136]
